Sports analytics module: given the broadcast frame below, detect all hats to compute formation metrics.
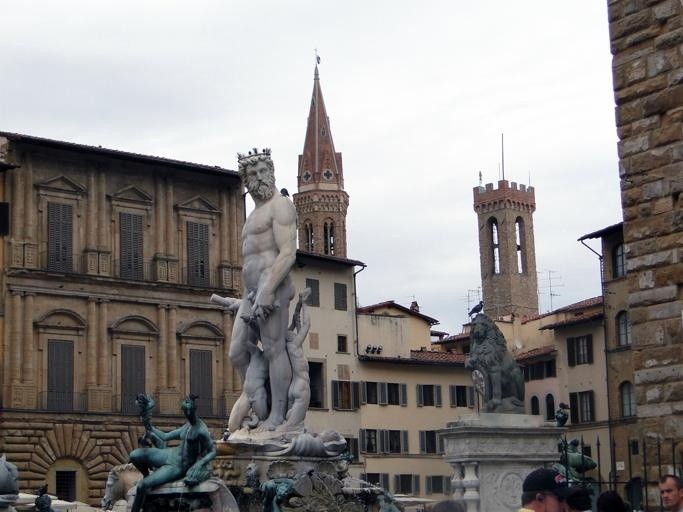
[523,468,581,498]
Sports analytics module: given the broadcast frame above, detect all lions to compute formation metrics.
[464,313,525,410]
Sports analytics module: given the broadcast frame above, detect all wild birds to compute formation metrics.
[468,301,484,317]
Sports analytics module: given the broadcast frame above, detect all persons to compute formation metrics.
[221,146,312,436]
[658,474,682,512]
[126,395,215,491]
[514,467,569,511]
[566,484,593,511]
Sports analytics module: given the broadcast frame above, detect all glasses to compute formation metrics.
[540,491,566,504]
